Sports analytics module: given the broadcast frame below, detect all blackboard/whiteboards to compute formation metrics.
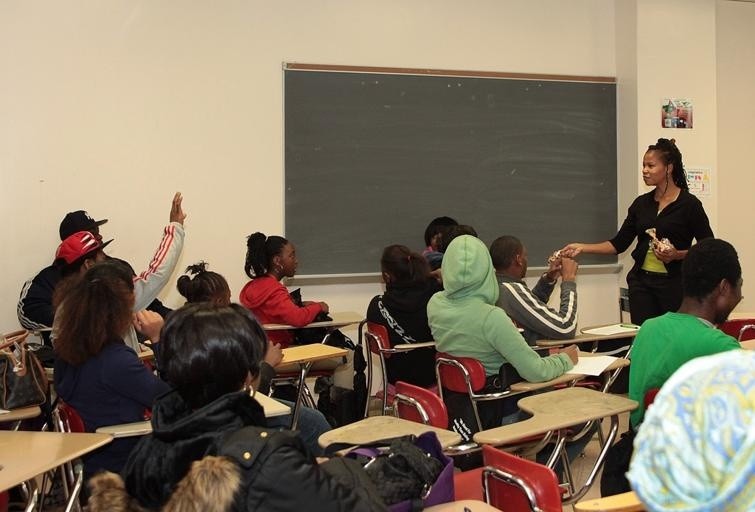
[282,63,623,289]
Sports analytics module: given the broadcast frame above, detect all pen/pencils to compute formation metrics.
[620,324,638,330]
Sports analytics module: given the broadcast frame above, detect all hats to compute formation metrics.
[60,210,107,242]
[55,231,113,266]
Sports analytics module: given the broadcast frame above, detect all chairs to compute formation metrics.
[52,399,87,512]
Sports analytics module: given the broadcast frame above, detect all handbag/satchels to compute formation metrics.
[1,329,48,410]
[354,431,453,512]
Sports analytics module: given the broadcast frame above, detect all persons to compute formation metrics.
[17,210,171,346]
[176,262,285,399]
[367,216,578,473]
[628,240,742,434]
[54,263,173,475]
[54,192,187,360]
[88,302,440,512]
[239,234,328,349]
[559,139,715,326]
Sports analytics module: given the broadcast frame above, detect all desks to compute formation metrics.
[0,429,111,511]
[0,405,42,426]
